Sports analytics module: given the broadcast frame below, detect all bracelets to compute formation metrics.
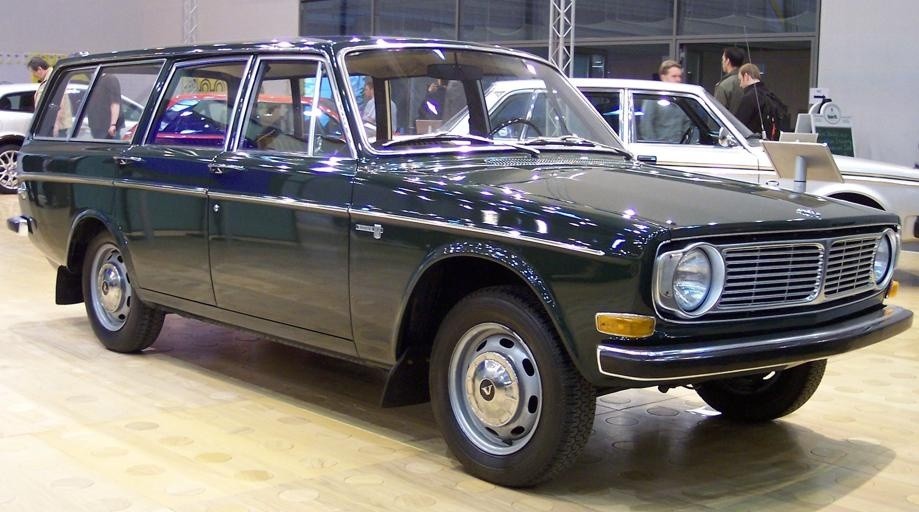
[109,122,116,126]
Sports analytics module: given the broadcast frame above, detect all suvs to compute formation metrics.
[4,30,917,494]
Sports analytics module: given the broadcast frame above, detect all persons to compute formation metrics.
[420,78,445,121]
[638,59,699,143]
[361,77,400,136]
[29,55,72,139]
[715,43,745,112]
[733,62,785,142]
[83,71,125,141]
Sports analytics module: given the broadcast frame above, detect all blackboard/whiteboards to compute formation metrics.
[815,126,854,157]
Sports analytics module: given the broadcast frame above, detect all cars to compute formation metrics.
[430,71,919,250]
[1,81,147,196]
[120,89,405,150]
